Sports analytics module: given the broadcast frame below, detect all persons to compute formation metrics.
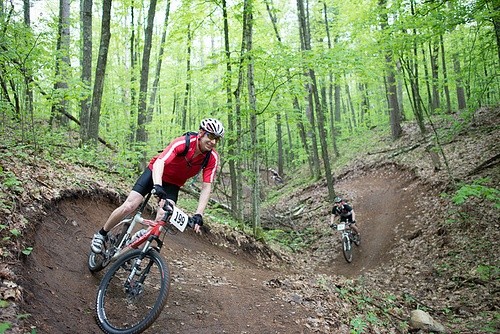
[90,118,226,254]
[329,197,360,237]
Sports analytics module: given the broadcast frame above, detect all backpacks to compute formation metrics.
[158,130,199,161]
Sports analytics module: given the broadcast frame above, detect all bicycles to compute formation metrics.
[332,219,361,263]
[87,187,201,334]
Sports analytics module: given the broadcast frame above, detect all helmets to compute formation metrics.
[335,197,341,202]
[200,118,224,139]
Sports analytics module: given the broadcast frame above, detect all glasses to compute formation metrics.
[206,132,219,143]
[335,202,340,204]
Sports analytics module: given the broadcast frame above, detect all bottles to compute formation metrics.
[131,229,146,242]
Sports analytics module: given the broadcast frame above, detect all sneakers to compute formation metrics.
[136,268,142,275]
[91,231,106,253]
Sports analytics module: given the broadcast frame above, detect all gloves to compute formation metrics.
[351,221,355,224]
[330,224,334,228]
